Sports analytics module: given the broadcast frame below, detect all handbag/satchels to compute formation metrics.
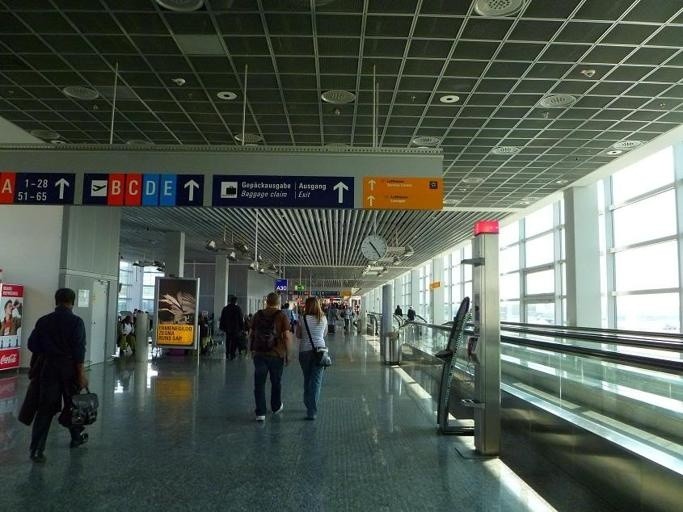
[313,345,332,367]
[56,385,102,427]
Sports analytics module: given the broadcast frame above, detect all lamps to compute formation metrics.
[132,252,179,278]
[207,225,284,278]
[363,244,418,276]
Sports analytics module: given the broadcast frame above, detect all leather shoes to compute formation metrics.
[30,448,46,463]
[68,432,89,448]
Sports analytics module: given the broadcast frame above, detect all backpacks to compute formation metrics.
[250,309,283,354]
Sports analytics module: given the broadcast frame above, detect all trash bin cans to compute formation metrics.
[384,333,402,365]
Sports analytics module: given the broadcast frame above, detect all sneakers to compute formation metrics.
[255,414,266,422]
[270,401,284,414]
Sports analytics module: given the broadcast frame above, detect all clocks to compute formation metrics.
[361,235,388,262]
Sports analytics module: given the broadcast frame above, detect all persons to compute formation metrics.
[118,301,359,356]
[394,305,403,318]
[295,295,329,422]
[218,295,246,361]
[406,306,416,321]
[25,287,89,464]
[0,299,22,336]
[248,293,290,423]
